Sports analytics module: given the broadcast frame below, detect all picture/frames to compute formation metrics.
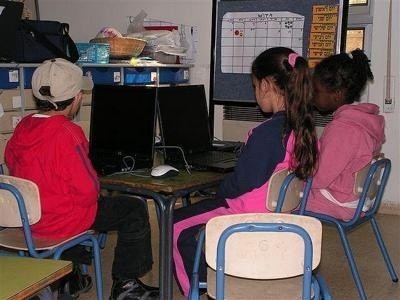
[0,255,73,300]
[99,150,233,300]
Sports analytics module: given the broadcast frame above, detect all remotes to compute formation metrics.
[150,164,180,178]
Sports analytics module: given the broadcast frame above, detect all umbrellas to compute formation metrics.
[109,278,160,299]
[58,274,93,300]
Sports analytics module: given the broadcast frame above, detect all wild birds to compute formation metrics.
[89,85,158,177]
[156,85,242,173]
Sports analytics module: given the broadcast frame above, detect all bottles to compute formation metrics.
[31,57,94,103]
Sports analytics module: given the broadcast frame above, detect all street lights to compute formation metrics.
[6,21,80,63]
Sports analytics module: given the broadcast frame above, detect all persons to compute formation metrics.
[4,58,160,300]
[171,48,319,300]
[292,49,385,220]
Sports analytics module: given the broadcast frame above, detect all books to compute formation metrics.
[0,68,191,175]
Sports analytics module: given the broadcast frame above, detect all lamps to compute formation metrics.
[205,213,323,300]
[187,168,333,300]
[0,174,104,300]
[304,153,398,300]
